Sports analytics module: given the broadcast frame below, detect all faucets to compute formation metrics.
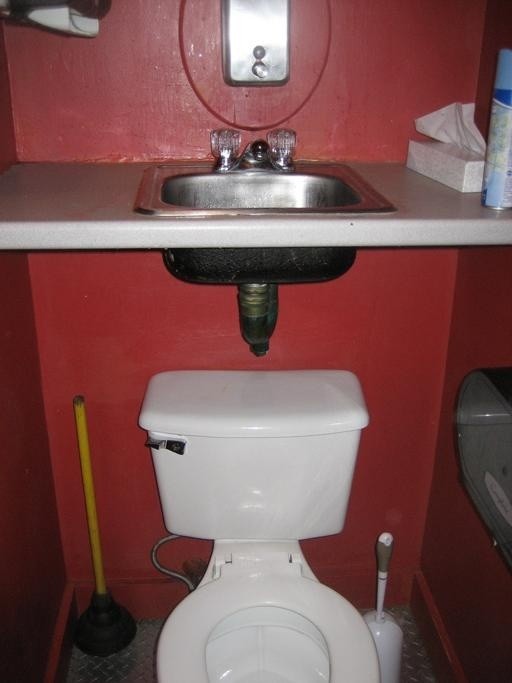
[239,137,273,169]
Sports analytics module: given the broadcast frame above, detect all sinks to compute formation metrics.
[132,160,393,213]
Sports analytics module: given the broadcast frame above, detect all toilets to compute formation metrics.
[143,370,382,683]
